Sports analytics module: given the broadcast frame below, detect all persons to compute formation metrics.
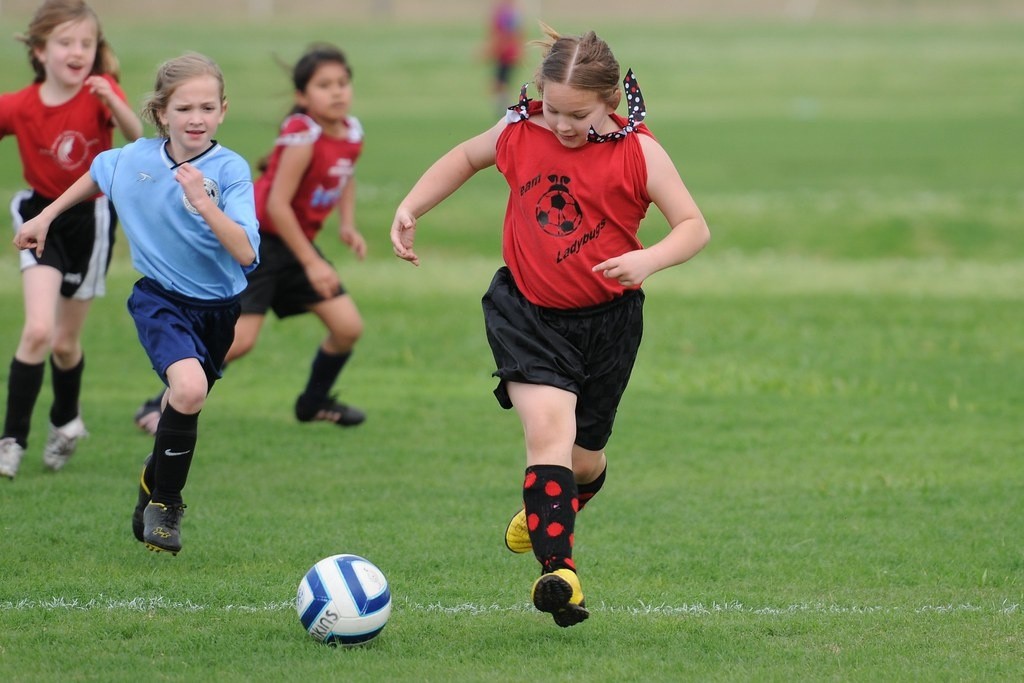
[0,0,368,556]
[389,19,711,629]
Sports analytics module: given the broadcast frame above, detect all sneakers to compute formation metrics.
[3,434,24,481]
[506,504,533,553]
[134,395,160,433]
[42,417,90,479]
[293,383,364,431]
[129,469,187,556]
[528,565,587,629]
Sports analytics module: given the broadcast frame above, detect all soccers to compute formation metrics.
[295,553,393,649]
